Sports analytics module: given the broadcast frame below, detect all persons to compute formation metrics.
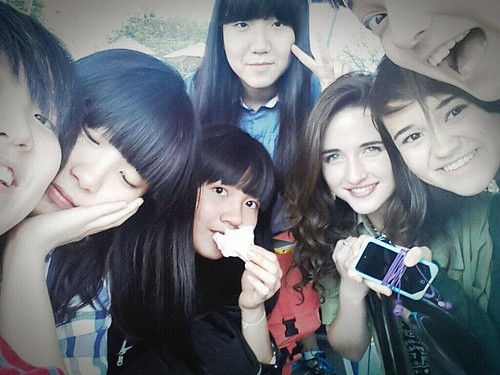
[0,0,500,375]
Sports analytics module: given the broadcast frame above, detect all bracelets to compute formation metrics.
[242,311,266,327]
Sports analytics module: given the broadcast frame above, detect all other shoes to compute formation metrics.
[300,350,333,374]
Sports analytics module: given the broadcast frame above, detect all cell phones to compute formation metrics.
[353,238,439,300]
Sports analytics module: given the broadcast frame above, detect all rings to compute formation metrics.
[342,241,349,247]
